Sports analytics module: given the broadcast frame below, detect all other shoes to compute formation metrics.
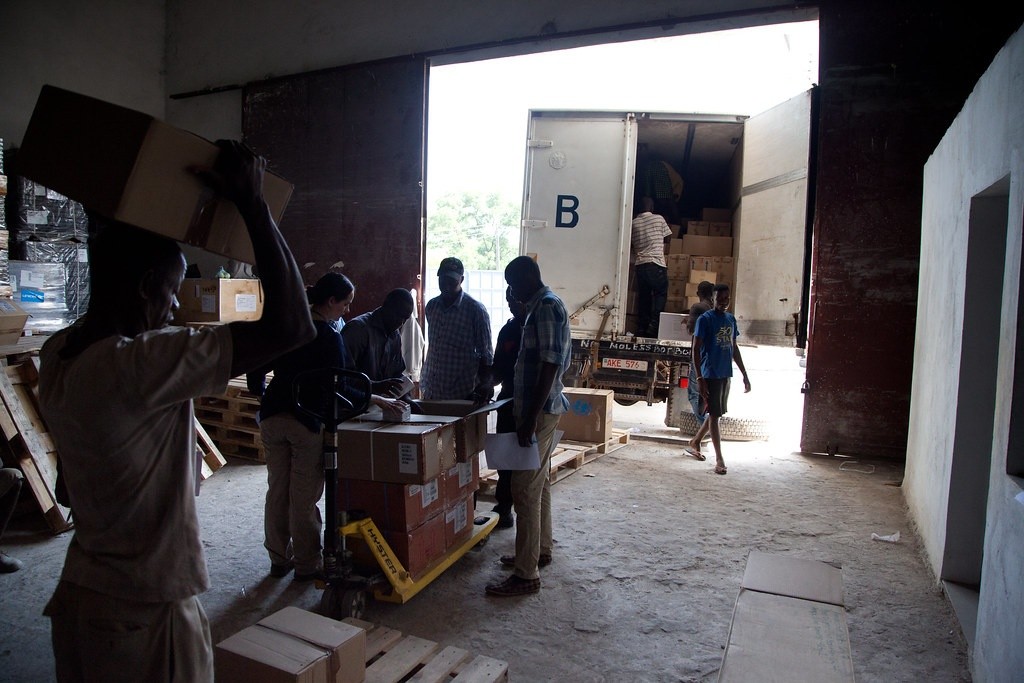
[0,548,22,573]
[270,555,295,577]
[474,508,513,528]
[638,322,647,335]
[294,566,323,582]
[647,327,657,337]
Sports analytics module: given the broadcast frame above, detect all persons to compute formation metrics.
[246,272,407,581]
[681,281,722,428]
[39,138,316,683]
[686,285,751,475]
[638,160,688,238]
[484,256,572,596]
[0,459,25,574]
[475,287,526,529]
[419,257,494,402]
[341,288,424,415]
[632,198,672,338]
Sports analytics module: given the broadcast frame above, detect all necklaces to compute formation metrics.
[312,309,327,322]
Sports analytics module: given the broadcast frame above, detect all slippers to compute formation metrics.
[686,447,706,460]
[716,464,727,474]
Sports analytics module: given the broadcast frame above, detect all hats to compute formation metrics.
[437,257,464,282]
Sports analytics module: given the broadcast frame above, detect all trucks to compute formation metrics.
[518,82,818,426]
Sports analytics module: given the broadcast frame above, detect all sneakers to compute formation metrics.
[501,554,552,565]
[485,575,540,595]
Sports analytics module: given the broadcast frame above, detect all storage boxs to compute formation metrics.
[335,397,514,579]
[15,84,294,263]
[0,299,28,345]
[169,278,264,328]
[555,387,614,442]
[626,208,734,335]
[216,606,366,682]
